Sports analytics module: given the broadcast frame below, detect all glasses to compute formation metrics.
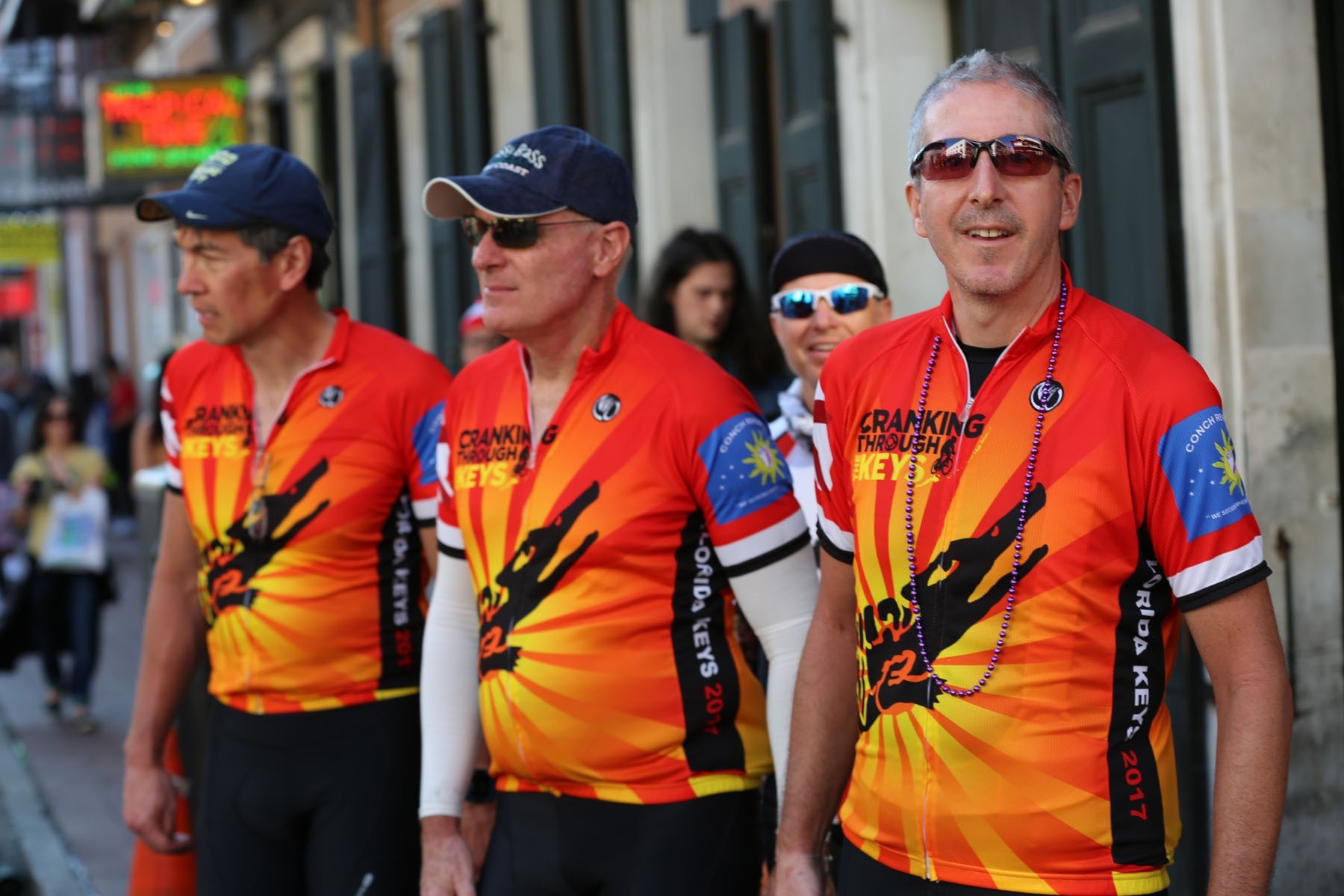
[461,214,598,248]
[770,281,885,320]
[911,134,1072,181]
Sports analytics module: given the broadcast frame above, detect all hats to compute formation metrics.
[769,230,889,299]
[136,144,328,225]
[422,124,637,226]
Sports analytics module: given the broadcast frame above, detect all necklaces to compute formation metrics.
[905,270,1068,699]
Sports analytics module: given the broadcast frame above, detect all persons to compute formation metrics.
[0,318,180,734]
[746,233,895,535]
[419,125,818,896]
[647,230,756,393]
[769,49,1291,896]
[122,144,497,896]
